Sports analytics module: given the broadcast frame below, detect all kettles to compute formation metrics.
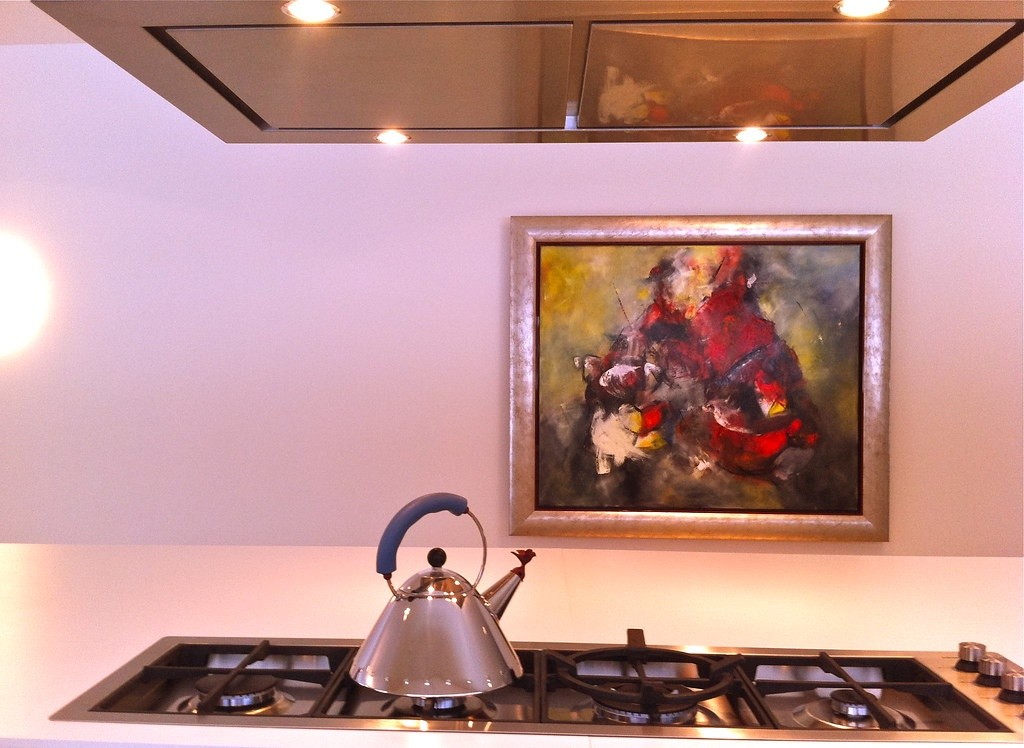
[348,492,534,699]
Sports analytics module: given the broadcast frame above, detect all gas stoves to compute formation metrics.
[51,637,1024,742]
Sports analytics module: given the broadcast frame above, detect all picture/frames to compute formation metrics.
[503,212,892,545]
